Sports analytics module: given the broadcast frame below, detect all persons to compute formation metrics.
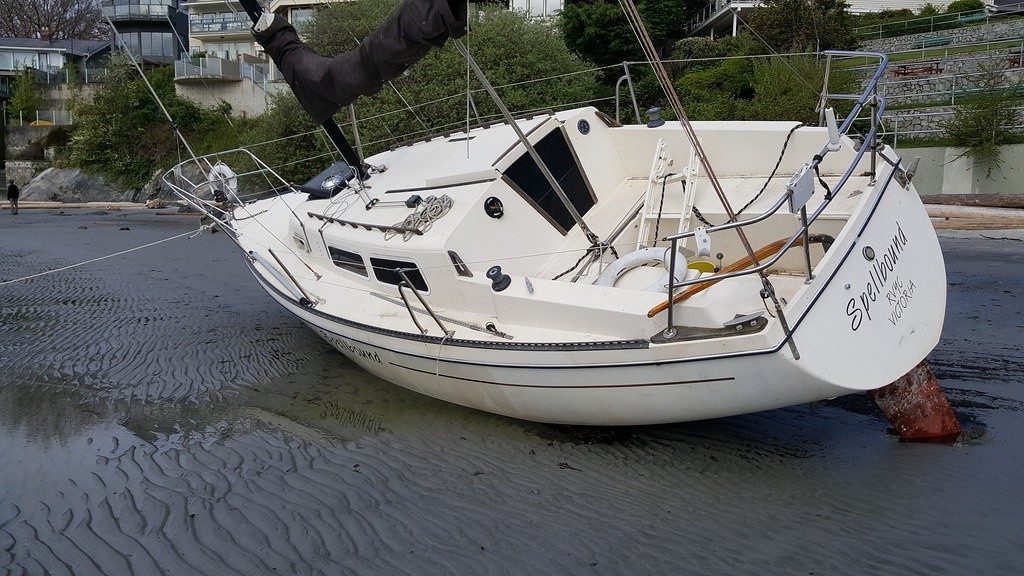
[7,179,19,215]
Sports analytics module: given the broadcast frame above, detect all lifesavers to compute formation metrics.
[598,246,688,294]
[209,165,238,201]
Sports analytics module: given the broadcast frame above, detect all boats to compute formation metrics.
[96,1,947,427]
